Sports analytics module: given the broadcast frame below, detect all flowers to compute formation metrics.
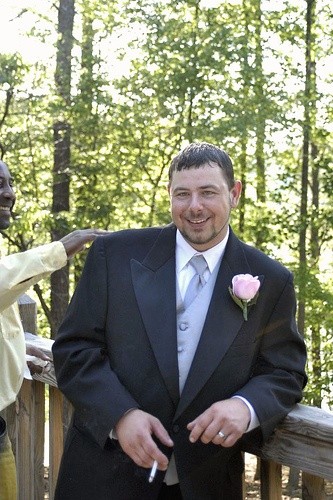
[228,274,264,322]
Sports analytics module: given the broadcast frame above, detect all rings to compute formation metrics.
[217,430,227,441]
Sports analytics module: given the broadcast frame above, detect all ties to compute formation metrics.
[183,255,208,313]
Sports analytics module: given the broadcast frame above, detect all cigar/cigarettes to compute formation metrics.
[149,459,158,482]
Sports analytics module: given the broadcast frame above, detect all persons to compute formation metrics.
[51,142,308,500]
[0,161,115,500]
